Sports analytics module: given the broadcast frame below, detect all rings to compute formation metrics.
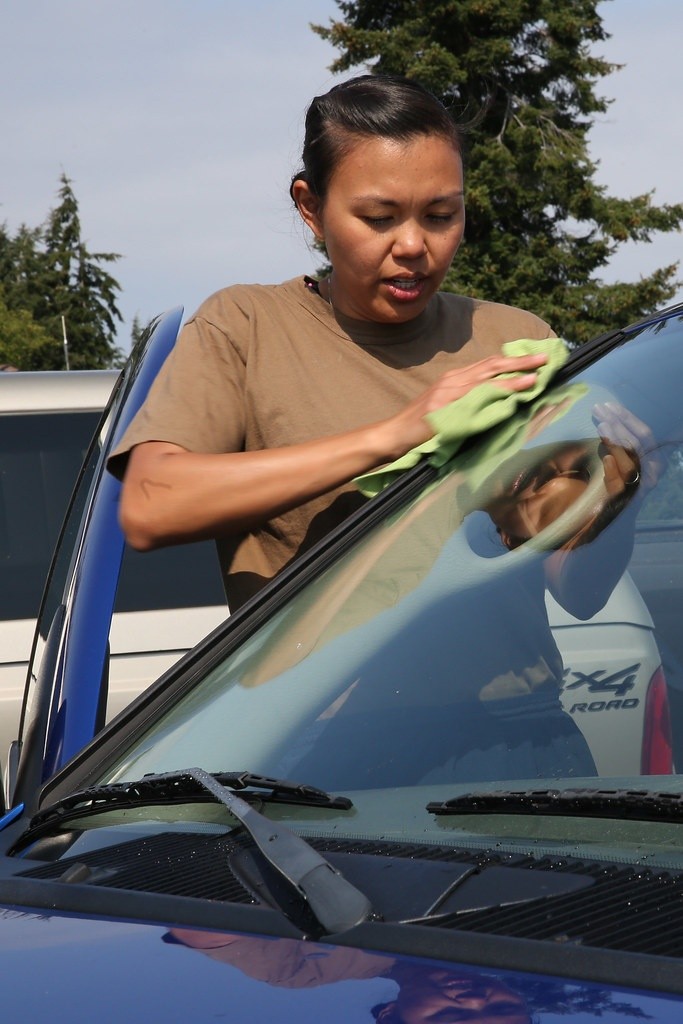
[625,471,641,486]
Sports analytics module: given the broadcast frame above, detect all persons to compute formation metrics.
[104,76,659,794]
[235,399,641,686]
[159,927,534,1024]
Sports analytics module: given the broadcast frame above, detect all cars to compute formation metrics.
[0,301,683,1024]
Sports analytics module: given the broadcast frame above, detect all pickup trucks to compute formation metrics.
[0,367,682,823]
